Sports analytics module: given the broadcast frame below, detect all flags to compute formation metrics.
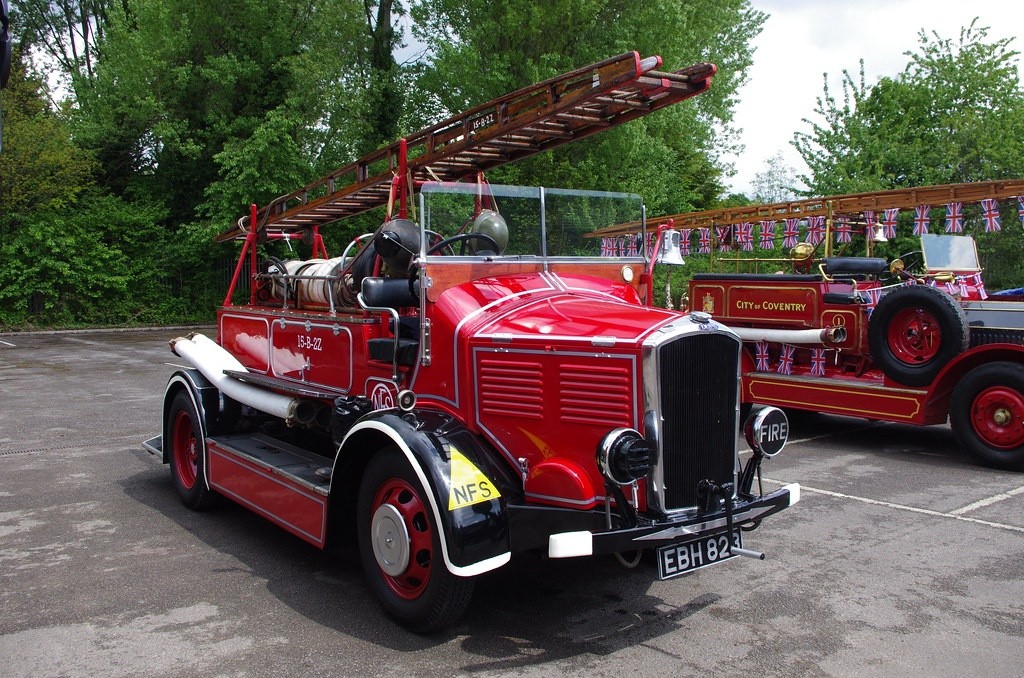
[810,349,826,376]
[945,203,963,233]
[600,238,607,257]
[883,208,899,239]
[913,205,931,236]
[973,273,989,300]
[782,218,800,247]
[946,281,959,295]
[866,289,881,322]
[626,236,638,257]
[805,215,825,245]
[958,275,969,298]
[758,221,776,250]
[754,342,769,372]
[645,232,653,256]
[836,216,852,244]
[776,344,795,375]
[617,237,625,257]
[901,281,917,287]
[679,230,691,256]
[980,199,1003,233]
[864,211,877,239]
[715,227,733,251]
[734,223,748,244]
[699,227,711,254]
[608,238,617,257]
[743,224,754,251]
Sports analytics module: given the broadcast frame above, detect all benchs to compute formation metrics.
[818,256,905,305]
[357,274,421,383]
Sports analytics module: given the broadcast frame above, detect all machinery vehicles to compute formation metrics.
[140,44,795,636]
[580,176,1024,473]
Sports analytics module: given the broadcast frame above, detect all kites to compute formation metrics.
[1016,196,1024,222]
